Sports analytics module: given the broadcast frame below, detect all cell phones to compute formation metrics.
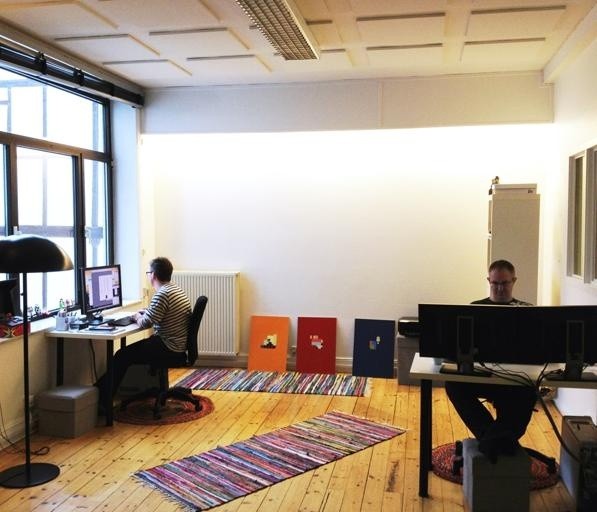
[90,326,115,331]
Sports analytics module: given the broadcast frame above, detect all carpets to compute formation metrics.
[431,440,561,491]
[175,363,373,400]
[130,408,405,511]
[112,394,214,427]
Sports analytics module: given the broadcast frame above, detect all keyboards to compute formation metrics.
[109,316,136,326]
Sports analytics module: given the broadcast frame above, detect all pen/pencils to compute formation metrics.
[67,312,78,316]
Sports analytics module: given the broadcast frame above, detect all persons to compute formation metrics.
[94,257,192,417]
[445,260,537,443]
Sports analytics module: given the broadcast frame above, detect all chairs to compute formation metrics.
[120,295,209,421]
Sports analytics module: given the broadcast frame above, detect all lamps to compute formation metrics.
[0,233,75,488]
[234,1,322,63]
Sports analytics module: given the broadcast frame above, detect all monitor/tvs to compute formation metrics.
[80,264,123,326]
[418,303,514,378]
[514,303,597,381]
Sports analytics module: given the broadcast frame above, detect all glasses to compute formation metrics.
[146,271,157,276]
[488,279,515,287]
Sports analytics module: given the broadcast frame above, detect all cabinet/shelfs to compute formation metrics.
[487,196,539,306]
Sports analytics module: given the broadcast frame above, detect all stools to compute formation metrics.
[34,385,100,439]
[459,434,530,511]
[393,331,445,388]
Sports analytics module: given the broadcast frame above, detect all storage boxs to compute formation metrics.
[491,182,538,195]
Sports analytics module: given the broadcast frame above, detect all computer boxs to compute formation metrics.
[560,416,597,512]
[119,360,162,395]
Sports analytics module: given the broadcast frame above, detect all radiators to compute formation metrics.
[169,270,242,360]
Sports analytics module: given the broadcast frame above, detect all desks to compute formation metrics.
[44,309,150,429]
[408,349,597,499]
